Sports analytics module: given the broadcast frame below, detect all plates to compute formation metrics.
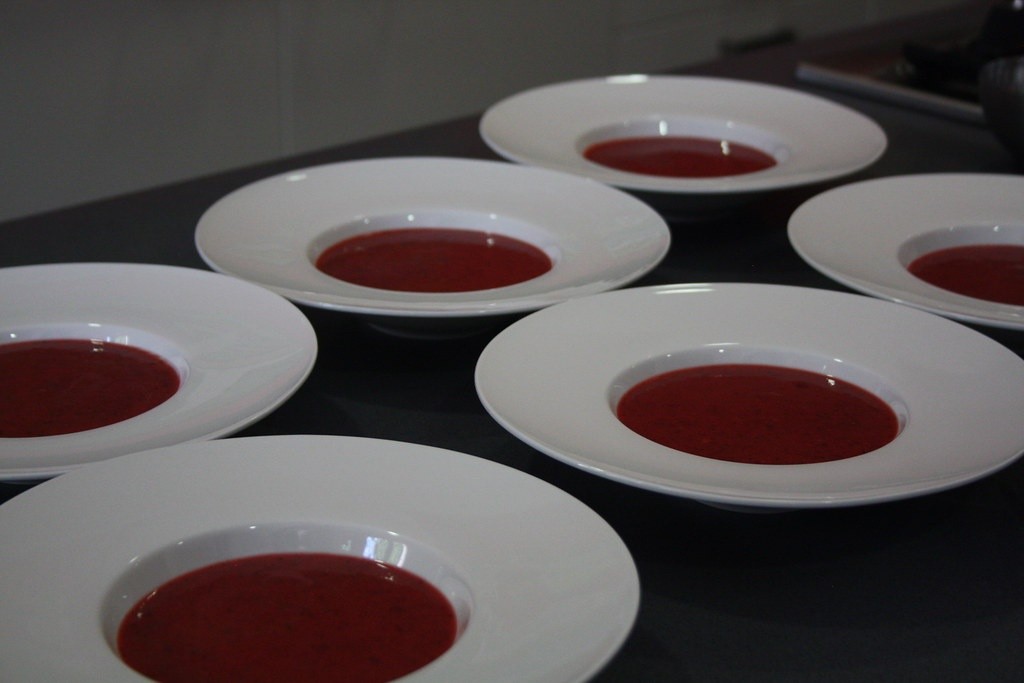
[195,157,671,334]
[790,174,1024,334]
[0,262,319,491]
[472,282,1024,513]
[0,433,642,683]
[480,74,886,196]
